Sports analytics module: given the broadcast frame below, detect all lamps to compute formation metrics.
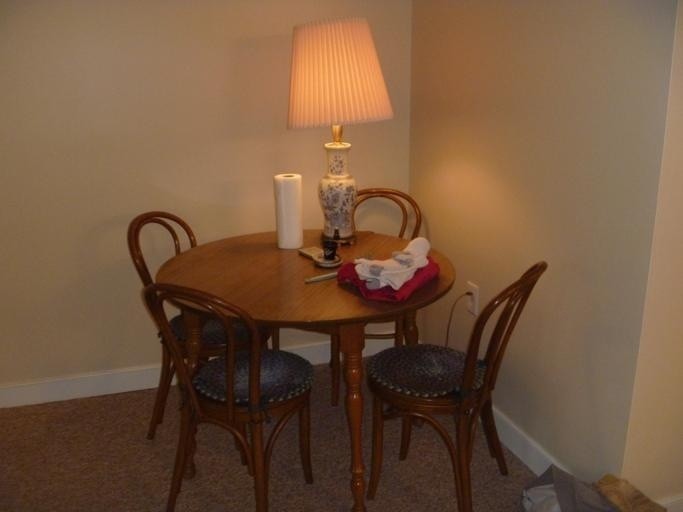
[285,16,394,241]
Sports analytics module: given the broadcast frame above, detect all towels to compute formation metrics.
[336,235,435,304]
[352,238,430,283]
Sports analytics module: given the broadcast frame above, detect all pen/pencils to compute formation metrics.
[304,272,338,284]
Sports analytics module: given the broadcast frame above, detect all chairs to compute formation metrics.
[325,187,425,409]
[140,278,314,512]
[128,210,279,467]
[366,260,548,510]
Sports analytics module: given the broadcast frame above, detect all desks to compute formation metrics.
[152,230,454,512]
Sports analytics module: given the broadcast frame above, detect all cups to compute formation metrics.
[321,236,339,258]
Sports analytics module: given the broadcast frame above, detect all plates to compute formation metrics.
[312,253,343,268]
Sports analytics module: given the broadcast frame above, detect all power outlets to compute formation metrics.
[460,282,478,315]
